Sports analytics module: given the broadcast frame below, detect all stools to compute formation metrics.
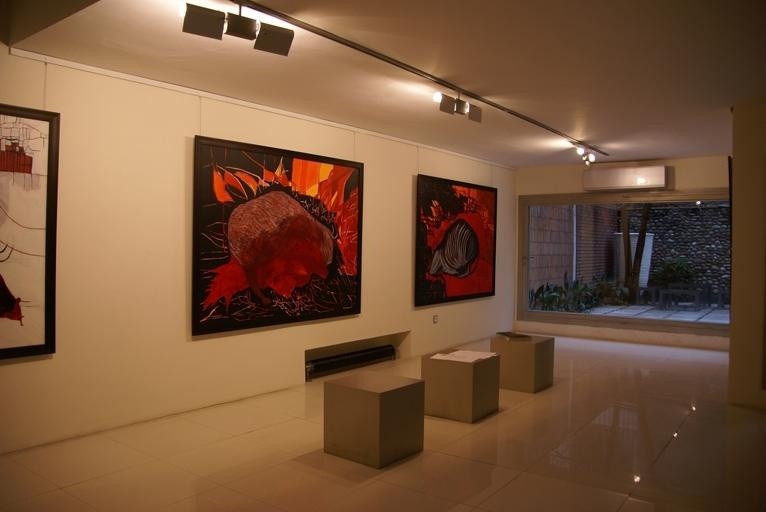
[637,283,726,309]
[323,330,555,470]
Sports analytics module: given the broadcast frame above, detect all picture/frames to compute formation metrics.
[191,134,364,342]
[1,104,61,360]
[414,173,497,306]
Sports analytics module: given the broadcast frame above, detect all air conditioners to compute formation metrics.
[583,166,665,192]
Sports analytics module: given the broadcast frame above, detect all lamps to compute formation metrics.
[437,93,481,125]
[570,137,595,166]
[180,0,295,60]
